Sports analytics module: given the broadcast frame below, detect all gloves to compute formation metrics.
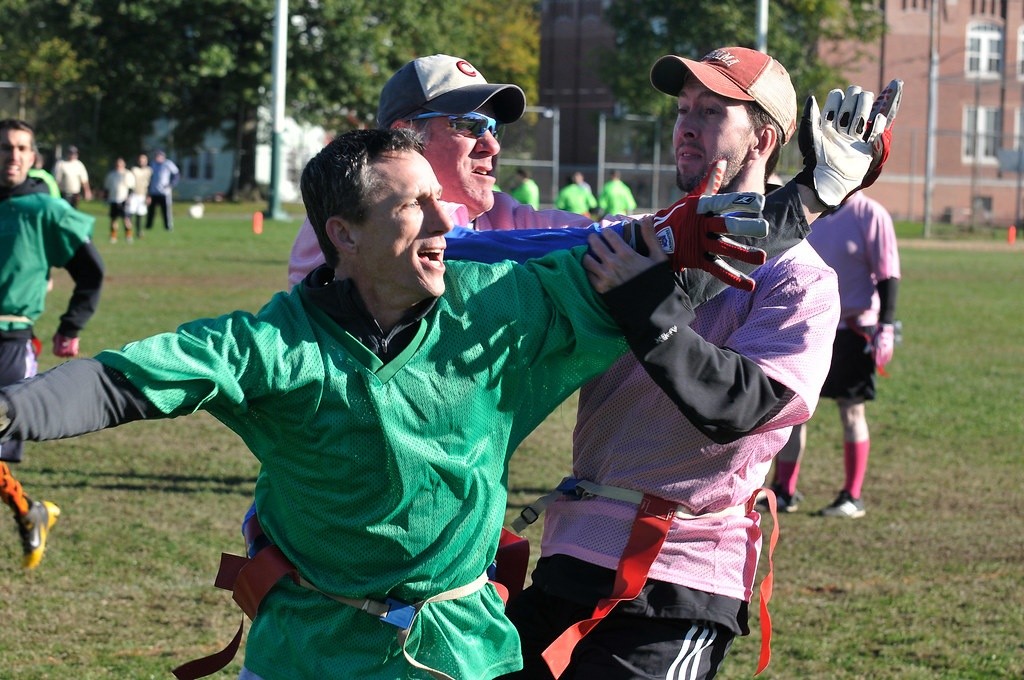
[53,334,79,357]
[794,78,904,210]
[631,157,769,292]
[871,323,895,369]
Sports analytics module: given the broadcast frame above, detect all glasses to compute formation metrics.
[407,112,495,139]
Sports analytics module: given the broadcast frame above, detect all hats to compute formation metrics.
[378,54,527,131]
[650,47,797,145]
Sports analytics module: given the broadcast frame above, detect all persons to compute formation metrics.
[0,119,105,571]
[34,146,91,209]
[286,55,769,291]
[513,167,540,211]
[104,151,179,242]
[514,47,843,680]
[555,170,636,220]
[754,192,900,518]
[0,80,904,680]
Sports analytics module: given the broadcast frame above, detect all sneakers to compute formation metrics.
[756,483,800,512]
[13,500,60,571]
[819,490,866,518]
